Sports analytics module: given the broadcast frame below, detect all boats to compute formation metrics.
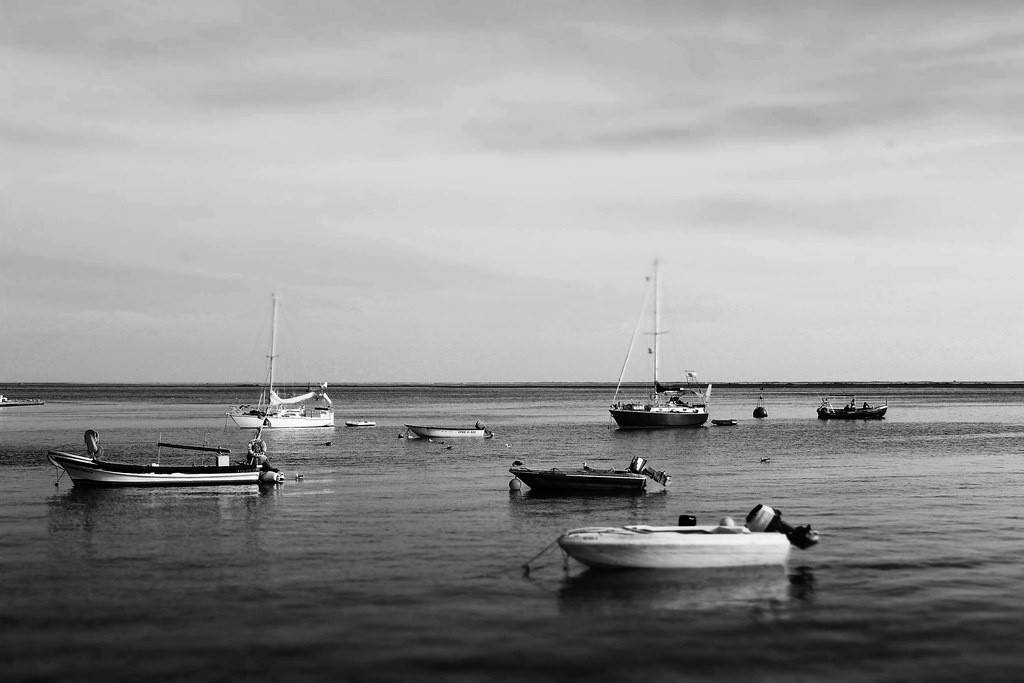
[711,419,738,427]
[46,431,258,489]
[817,398,888,418]
[559,505,821,569]
[508,459,670,492]
[406,423,487,438]
[346,421,375,426]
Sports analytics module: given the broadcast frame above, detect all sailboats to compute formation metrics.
[228,293,336,431]
[606,259,712,430]
